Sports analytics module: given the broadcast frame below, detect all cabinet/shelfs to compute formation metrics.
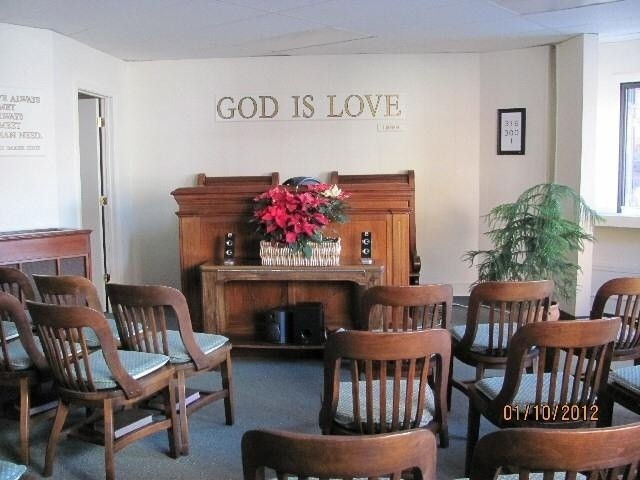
[0,225,98,330]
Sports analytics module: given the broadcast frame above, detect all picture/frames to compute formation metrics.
[494,103,527,157]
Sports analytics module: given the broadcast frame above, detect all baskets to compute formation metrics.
[258,239,341,266]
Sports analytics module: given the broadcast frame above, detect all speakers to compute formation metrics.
[359,232,372,265]
[293,302,327,345]
[263,305,295,344]
[224,232,235,266]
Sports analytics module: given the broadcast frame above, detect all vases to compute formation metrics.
[258,234,343,268]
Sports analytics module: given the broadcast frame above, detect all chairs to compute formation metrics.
[464,317,621,479]
[544,277,640,373]
[470,422,639,479]
[358,283,451,449]
[319,330,451,449]
[25,300,181,479]
[105,283,235,456]
[0,265,156,465]
[604,365,640,428]
[241,428,437,480]
[446,280,554,412]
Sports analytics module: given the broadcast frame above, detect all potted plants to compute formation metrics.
[460,178,612,338]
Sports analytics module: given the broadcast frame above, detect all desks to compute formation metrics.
[197,256,388,355]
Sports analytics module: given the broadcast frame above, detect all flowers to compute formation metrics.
[236,179,353,264]
